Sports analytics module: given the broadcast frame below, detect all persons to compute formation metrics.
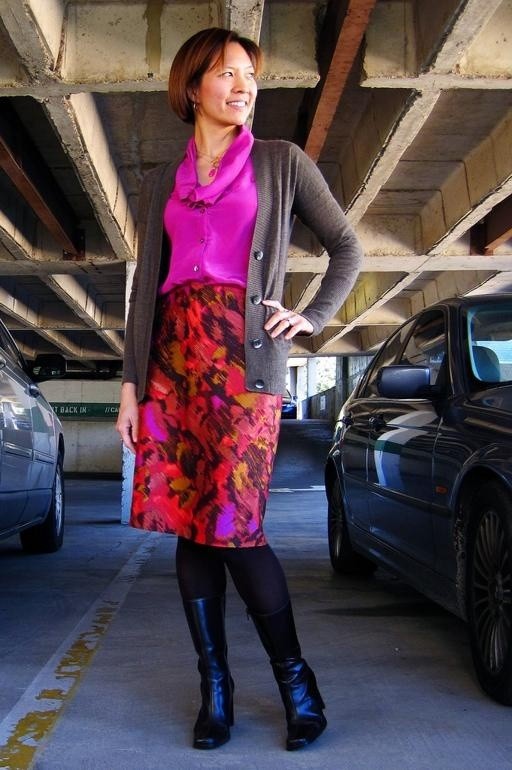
[114,27,365,751]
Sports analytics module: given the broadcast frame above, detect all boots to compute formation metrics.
[182,594,234,749]
[245,598,327,750]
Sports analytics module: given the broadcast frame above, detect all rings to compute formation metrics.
[284,318,291,327]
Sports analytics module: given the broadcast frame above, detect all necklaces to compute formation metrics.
[196,146,227,178]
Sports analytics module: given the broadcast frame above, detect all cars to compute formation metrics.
[0,318,66,555]
[322,293,509,678]
[281,388,298,419]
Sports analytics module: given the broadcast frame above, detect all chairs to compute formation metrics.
[432,346,499,387]
